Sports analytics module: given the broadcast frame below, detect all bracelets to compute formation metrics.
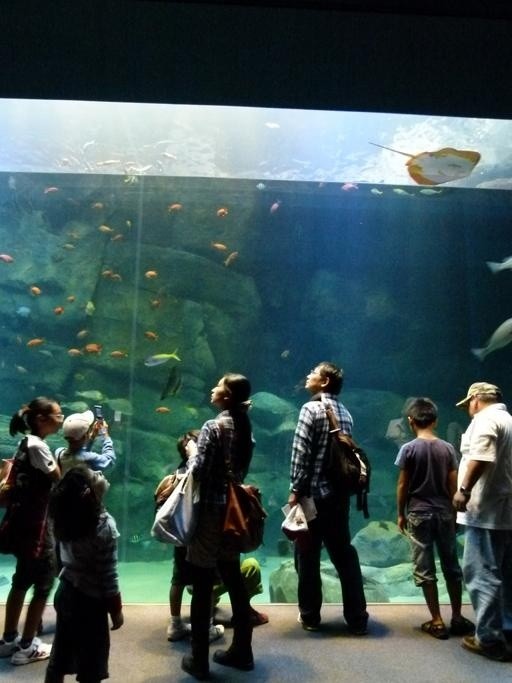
[289,486,300,495]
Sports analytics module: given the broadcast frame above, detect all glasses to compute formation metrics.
[50,414,64,420]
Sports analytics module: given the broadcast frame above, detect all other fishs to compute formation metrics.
[0,105,443,555]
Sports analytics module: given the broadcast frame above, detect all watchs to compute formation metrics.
[459,483,471,495]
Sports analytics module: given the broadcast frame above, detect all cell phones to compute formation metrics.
[96,407,103,427]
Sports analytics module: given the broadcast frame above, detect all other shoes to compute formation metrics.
[208,624,225,642]
[450,615,476,635]
[167,622,191,640]
[182,654,199,677]
[213,649,254,671]
[344,616,369,635]
[297,612,317,630]
[462,636,481,654]
[421,620,448,640]
[231,613,268,628]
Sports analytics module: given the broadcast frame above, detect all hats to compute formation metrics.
[455,382,502,408]
[63,410,94,440]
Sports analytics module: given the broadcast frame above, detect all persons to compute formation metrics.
[180,373,256,682]
[0,396,64,666]
[184,556,269,629]
[453,380,512,656]
[165,430,226,645]
[281,359,369,635]
[53,409,117,576]
[44,462,124,683]
[393,396,476,641]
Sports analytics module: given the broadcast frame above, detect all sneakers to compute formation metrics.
[2,635,21,656]
[10,637,53,664]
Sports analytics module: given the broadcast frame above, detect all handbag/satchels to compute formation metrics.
[221,480,266,552]
[0,459,17,504]
[324,428,369,495]
[151,471,202,547]
